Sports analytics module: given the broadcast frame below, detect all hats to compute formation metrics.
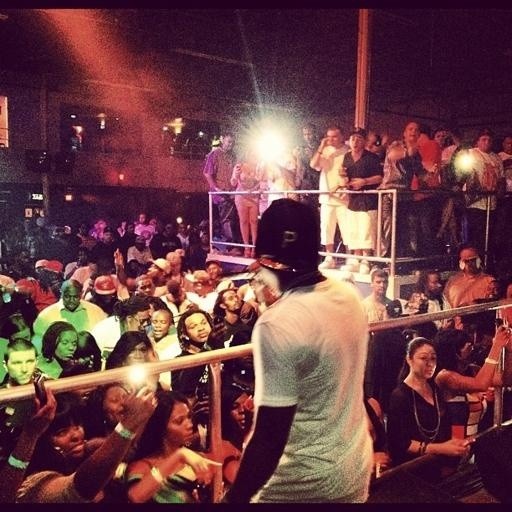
[349,128,367,138]
[149,257,171,275]
[95,276,116,295]
[205,260,222,268]
[103,226,114,232]
[255,198,320,270]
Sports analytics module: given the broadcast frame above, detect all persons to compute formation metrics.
[70,125,94,152]
[217,198,372,502]
[160,126,210,162]
[1,207,279,504]
[203,121,512,274]
[362,248,512,503]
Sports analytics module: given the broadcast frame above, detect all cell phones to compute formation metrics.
[33,373,48,406]
[495,319,504,330]
[461,439,477,446]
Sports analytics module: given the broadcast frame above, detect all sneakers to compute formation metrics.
[318,257,370,273]
